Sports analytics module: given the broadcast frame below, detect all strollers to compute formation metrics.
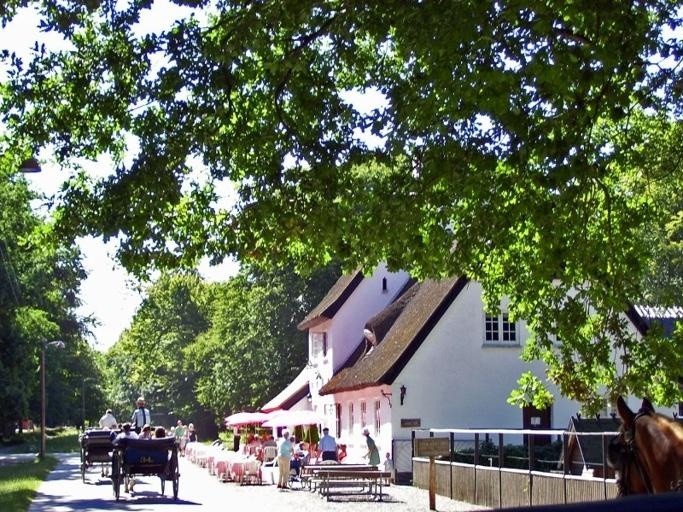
[288,449,309,489]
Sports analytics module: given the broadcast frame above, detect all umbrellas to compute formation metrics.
[224,409,334,427]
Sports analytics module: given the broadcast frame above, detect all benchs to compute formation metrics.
[80,429,176,476]
[301,474,390,502]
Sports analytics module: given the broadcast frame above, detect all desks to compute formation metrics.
[303,465,391,502]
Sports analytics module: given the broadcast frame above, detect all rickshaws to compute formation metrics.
[78,424,181,500]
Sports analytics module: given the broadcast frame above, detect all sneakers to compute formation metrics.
[276,485,290,489]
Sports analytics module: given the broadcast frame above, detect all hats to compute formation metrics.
[136,396,146,405]
[361,428,371,436]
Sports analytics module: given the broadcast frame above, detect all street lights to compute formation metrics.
[82,376,96,431]
[37,340,66,458]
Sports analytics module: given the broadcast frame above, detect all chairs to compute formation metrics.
[185,434,347,486]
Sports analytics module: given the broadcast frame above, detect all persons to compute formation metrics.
[97,396,198,453]
[320,428,339,461]
[244,428,317,488]
[362,429,380,465]
[234,424,241,451]
[384,453,394,486]
